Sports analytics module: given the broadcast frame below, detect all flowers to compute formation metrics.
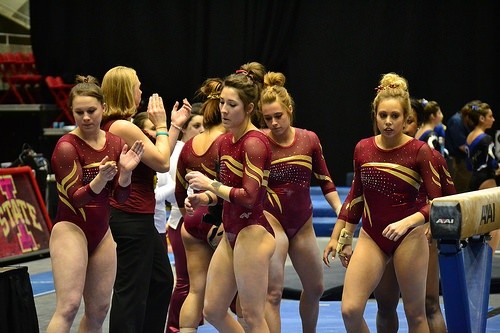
[375,85,397,91]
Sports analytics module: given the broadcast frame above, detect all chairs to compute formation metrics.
[0,53,77,125]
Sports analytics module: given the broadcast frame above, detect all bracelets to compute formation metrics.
[154,126,168,130]
[156,131,169,138]
[211,179,222,195]
[338,228,353,245]
[170,122,183,131]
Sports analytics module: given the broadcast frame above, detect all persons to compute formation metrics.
[132,103,238,333]
[175,78,249,333]
[336,72,458,333]
[46,75,145,333]
[18,143,48,206]
[100,65,192,333]
[185,62,276,333]
[257,71,343,333]
[323,99,500,333]
